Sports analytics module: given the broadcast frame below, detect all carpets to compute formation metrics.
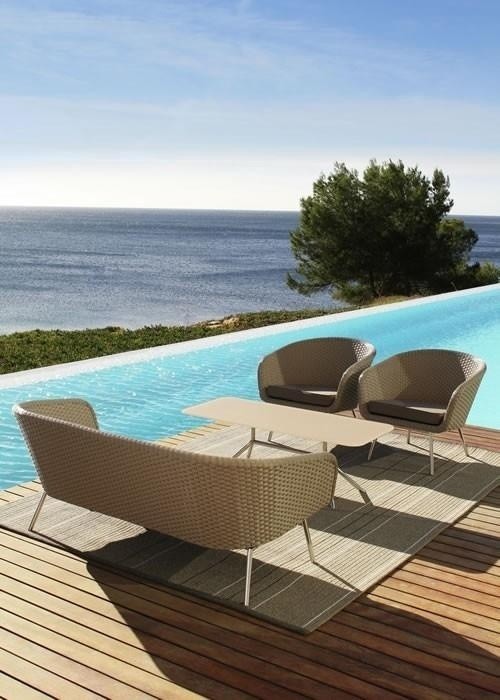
[0,426,500,635]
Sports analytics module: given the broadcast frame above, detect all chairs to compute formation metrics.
[257,337,376,452]
[358,349,487,475]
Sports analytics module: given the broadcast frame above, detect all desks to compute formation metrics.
[181,396,395,511]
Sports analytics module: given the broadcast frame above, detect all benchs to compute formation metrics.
[12,397,339,607]
